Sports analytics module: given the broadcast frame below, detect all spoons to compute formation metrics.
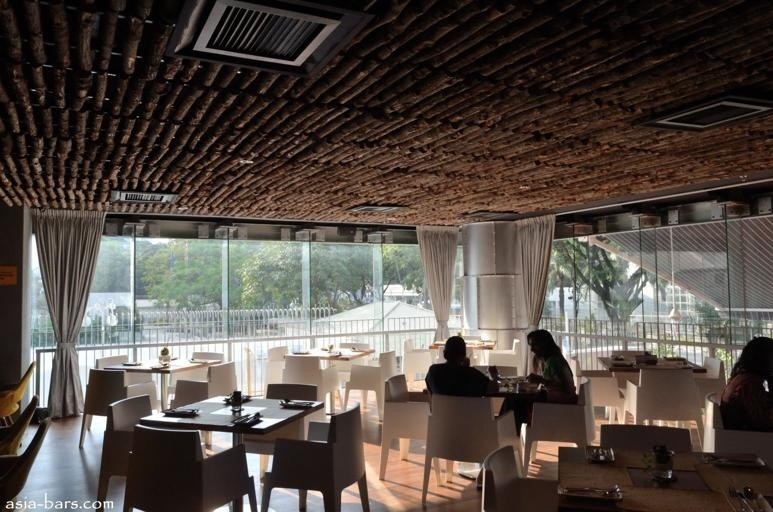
[743,486,764,511]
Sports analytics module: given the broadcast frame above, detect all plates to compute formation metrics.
[612,360,634,367]
[231,414,262,426]
[122,362,140,366]
[584,445,615,464]
[557,483,622,502]
[161,408,202,417]
[291,351,308,354]
[280,402,314,408]
[149,365,168,368]
[709,453,766,470]
[223,395,251,404]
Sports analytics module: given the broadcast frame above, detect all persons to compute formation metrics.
[499,329,578,438]
[718,337,772,436]
[424,336,499,400]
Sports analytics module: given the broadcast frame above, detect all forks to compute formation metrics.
[728,486,753,512]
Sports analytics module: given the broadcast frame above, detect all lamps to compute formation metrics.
[666,227,680,319]
